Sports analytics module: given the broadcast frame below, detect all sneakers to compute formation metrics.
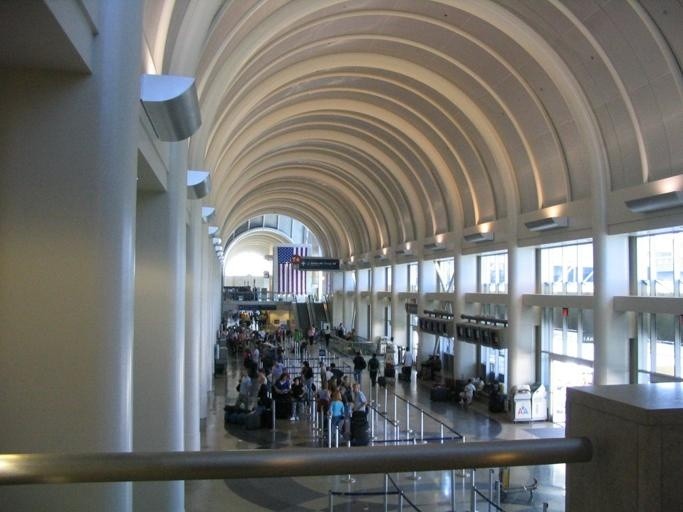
[288,416,300,422]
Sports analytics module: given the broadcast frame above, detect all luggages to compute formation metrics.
[488,393,502,412]
[378,370,386,388]
[351,411,370,447]
[430,385,451,401]
[344,417,350,439]
[260,409,274,430]
[384,360,396,385]
[243,352,251,368]
[276,394,291,419]
[398,367,411,381]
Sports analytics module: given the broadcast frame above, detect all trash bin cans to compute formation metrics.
[385,344,406,365]
[377,336,386,353]
[219,346,228,360]
[510,382,548,425]
[421,364,432,380]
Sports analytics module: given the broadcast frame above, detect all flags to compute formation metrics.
[278,247,307,295]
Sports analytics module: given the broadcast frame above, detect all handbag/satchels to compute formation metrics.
[236,376,243,392]
[311,384,316,392]
[224,405,251,424]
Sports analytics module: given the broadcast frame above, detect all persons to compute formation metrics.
[368,353,380,387]
[421,355,441,371]
[221,309,369,447]
[402,347,413,382]
[464,378,476,406]
[386,336,394,345]
[494,382,505,403]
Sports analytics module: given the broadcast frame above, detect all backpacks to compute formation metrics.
[360,357,366,369]
